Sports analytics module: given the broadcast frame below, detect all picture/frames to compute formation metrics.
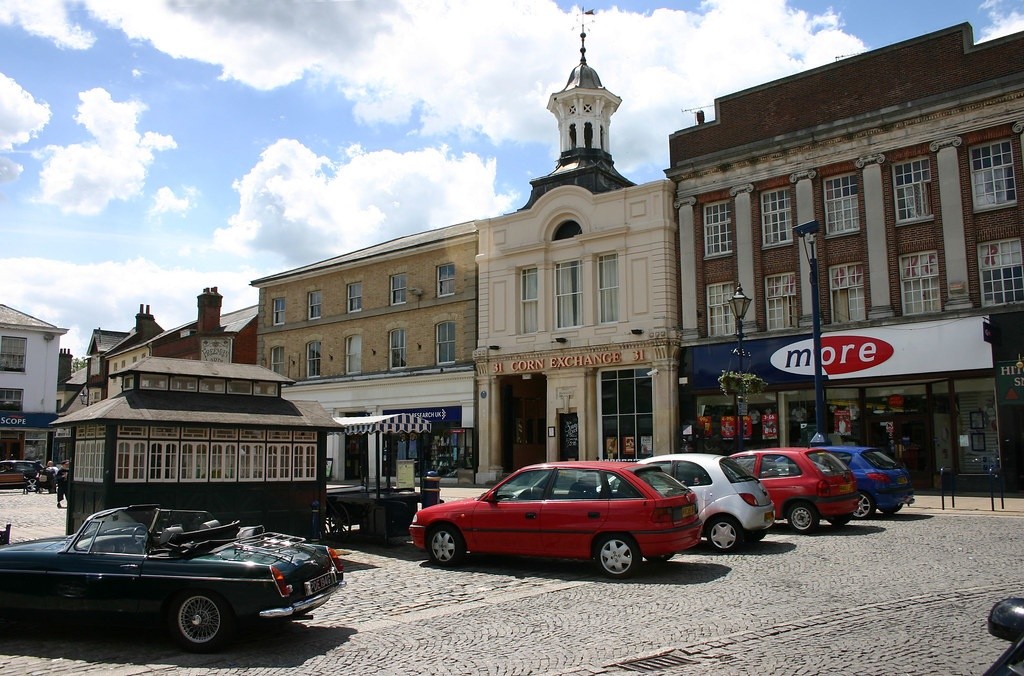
[971,432,986,451]
[970,411,985,429]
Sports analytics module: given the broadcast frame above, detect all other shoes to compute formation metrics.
[56,502,61,507]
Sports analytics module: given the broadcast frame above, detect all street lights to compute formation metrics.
[728,283,752,452]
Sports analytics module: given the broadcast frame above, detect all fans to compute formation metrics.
[844,405,860,420]
[792,407,807,422]
[748,410,760,424]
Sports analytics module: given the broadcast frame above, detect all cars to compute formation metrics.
[1,504,348,654]
[409,461,705,579]
[728,447,859,533]
[595,453,775,552]
[774,446,916,519]
[0,460,44,493]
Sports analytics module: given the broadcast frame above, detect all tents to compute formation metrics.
[326,413,433,549]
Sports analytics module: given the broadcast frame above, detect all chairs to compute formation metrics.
[199,520,220,530]
[514,486,545,499]
[158,527,186,551]
[567,476,588,498]
[610,481,632,498]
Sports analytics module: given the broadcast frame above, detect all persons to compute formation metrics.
[2,460,70,508]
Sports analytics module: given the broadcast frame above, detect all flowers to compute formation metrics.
[717,369,768,403]
